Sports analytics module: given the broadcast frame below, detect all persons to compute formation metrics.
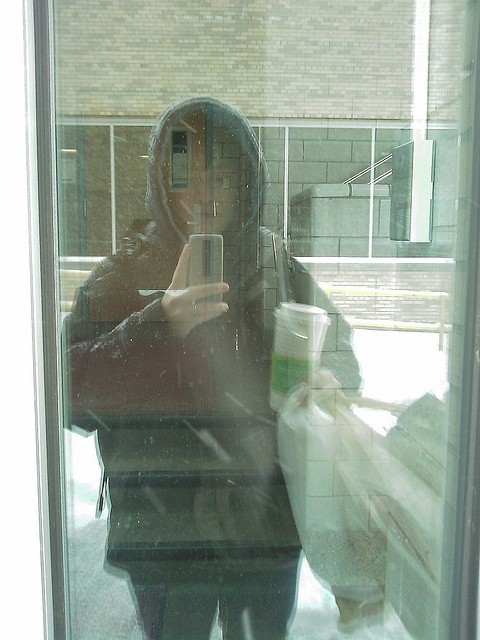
[56,97,362,639]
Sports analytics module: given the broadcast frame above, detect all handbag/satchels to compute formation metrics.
[197,455,300,575]
[332,400,443,639]
[332,392,451,497]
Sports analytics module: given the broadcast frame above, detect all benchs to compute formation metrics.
[328,287,452,351]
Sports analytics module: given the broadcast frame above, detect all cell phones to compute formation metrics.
[188,234,224,303]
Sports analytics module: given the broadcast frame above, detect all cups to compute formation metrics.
[269,301,331,413]
